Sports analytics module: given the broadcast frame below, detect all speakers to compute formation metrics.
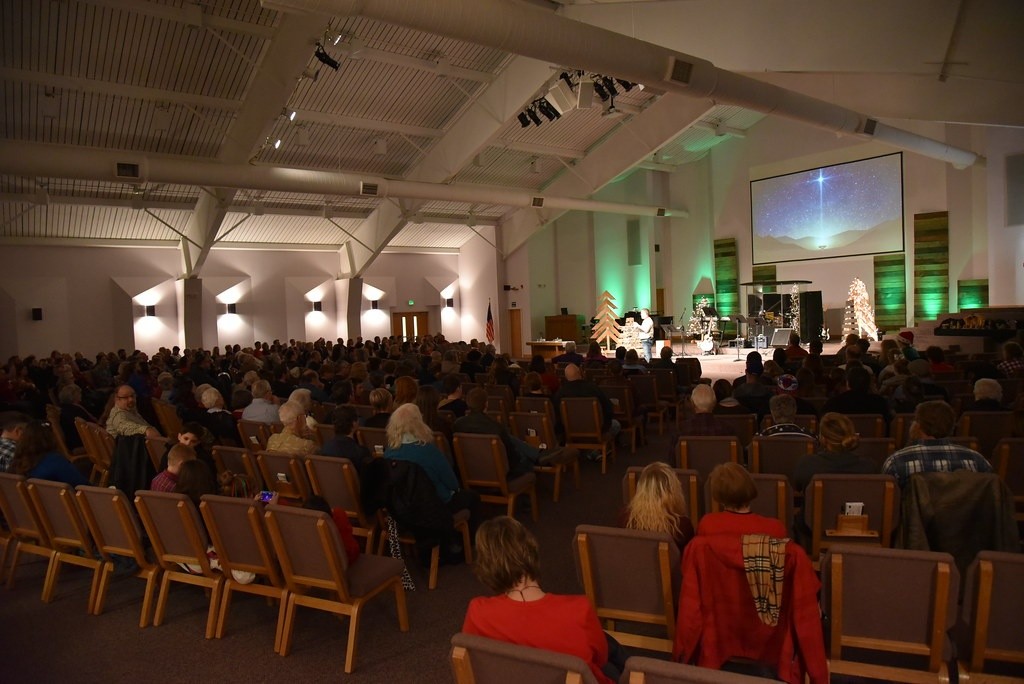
[770,328,796,349]
[544,76,667,115]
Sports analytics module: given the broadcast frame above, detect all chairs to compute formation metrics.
[0,352,1024,684]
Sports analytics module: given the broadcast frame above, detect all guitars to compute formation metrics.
[819,304,830,340]
[700,313,714,352]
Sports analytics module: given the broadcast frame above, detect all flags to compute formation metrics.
[486,304,494,343]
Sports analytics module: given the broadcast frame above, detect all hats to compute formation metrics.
[896,331,914,346]
[747,360,763,376]
[776,374,798,393]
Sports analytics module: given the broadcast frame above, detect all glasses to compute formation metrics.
[299,413,307,418]
[117,393,137,400]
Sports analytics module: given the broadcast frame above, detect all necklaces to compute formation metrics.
[509,585,541,602]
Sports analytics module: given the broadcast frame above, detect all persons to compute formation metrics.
[624,331,1024,620]
[460,516,628,684]
[0,334,646,578]
[963,315,984,329]
[634,308,654,363]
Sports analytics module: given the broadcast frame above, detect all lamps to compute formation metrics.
[518,70,637,129]
[372,301,379,309]
[446,299,453,307]
[146,306,155,316]
[325,27,342,45]
[315,45,341,73]
[283,109,297,122]
[314,301,322,312]
[31,307,42,320]
[267,137,281,150]
[228,304,236,313]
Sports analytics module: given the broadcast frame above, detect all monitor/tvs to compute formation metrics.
[561,308,568,314]
